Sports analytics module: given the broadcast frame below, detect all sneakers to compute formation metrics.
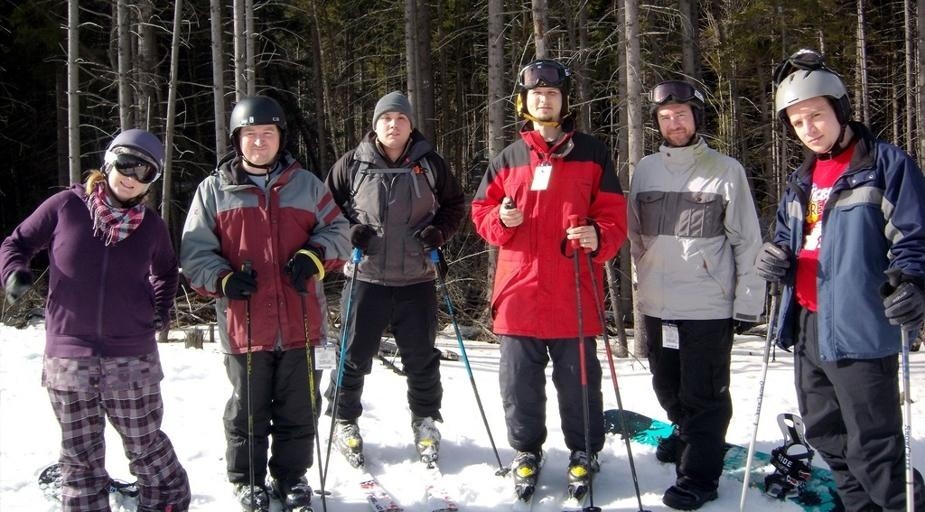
[411,411,443,464]
[662,473,720,512]
[567,447,602,500]
[271,472,313,508]
[512,445,543,502]
[232,480,270,511]
[333,417,364,451]
[656,425,689,465]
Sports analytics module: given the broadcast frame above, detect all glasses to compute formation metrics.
[112,154,159,184]
[517,62,564,88]
[772,52,825,89]
[648,80,695,104]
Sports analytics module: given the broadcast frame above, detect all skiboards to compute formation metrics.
[509,446,597,512]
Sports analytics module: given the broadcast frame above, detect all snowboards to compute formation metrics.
[600,409,851,511]
[38,458,146,511]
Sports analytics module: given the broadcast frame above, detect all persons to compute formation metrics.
[471,60,628,484]
[751,49,925,512]
[178,96,353,512]
[323,89,466,451]
[0,131,191,512]
[628,82,766,511]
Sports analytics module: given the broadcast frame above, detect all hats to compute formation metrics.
[371,90,416,136]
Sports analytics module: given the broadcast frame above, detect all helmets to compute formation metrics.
[649,80,705,129]
[105,129,167,170]
[228,94,288,145]
[774,69,854,140]
[517,58,569,108]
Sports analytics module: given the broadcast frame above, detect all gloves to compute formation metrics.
[348,221,377,252]
[878,277,925,330]
[3,269,35,306]
[754,240,793,282]
[288,251,322,296]
[732,317,759,336]
[221,271,258,301]
[411,224,439,252]
